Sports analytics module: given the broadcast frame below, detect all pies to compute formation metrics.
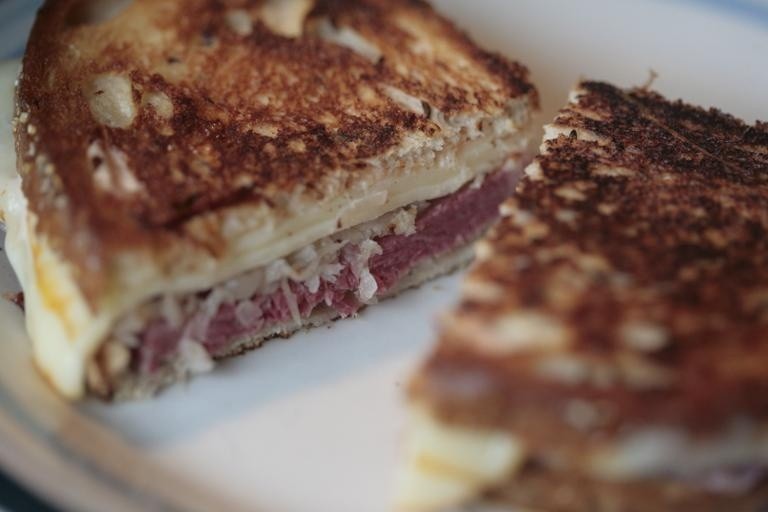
[2,1,544,406]
[393,72,768,511]
